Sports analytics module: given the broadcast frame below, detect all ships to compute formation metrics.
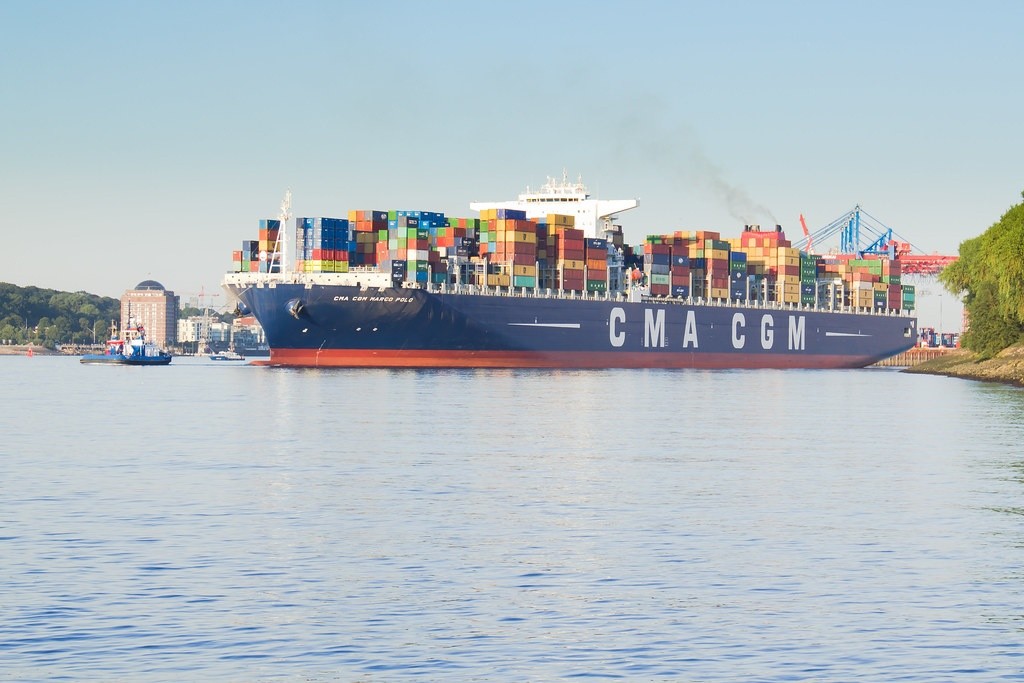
[223,169,917,367]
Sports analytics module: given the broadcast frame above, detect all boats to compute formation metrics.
[208,343,245,360]
[80,300,172,365]
